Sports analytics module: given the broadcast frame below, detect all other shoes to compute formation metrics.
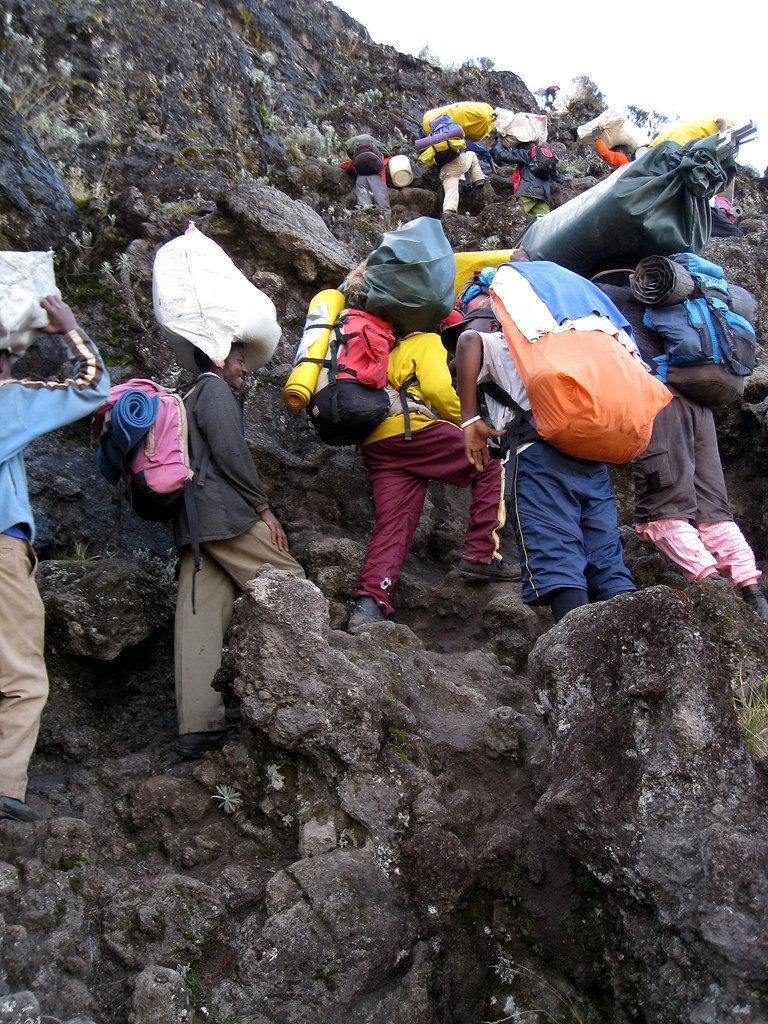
[1,793,43,823]
[175,732,225,758]
[744,592,767,620]
[460,553,524,582]
[348,600,383,632]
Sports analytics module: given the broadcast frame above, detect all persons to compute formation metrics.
[339,152,394,226]
[167,337,307,755]
[344,322,524,631]
[456,296,640,626]
[592,126,632,168]
[433,138,501,215]
[494,137,573,214]
[544,85,560,111]
[510,245,768,619]
[0,294,112,823]
[711,196,745,238]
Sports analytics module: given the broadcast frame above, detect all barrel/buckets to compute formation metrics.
[389,155,413,187]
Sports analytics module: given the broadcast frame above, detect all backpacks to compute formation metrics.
[306,307,420,445]
[93,371,226,523]
[643,250,759,405]
[530,144,558,182]
[430,122,461,165]
[476,260,673,464]
[354,139,383,175]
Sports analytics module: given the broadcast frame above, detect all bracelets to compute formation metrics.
[460,415,481,429]
